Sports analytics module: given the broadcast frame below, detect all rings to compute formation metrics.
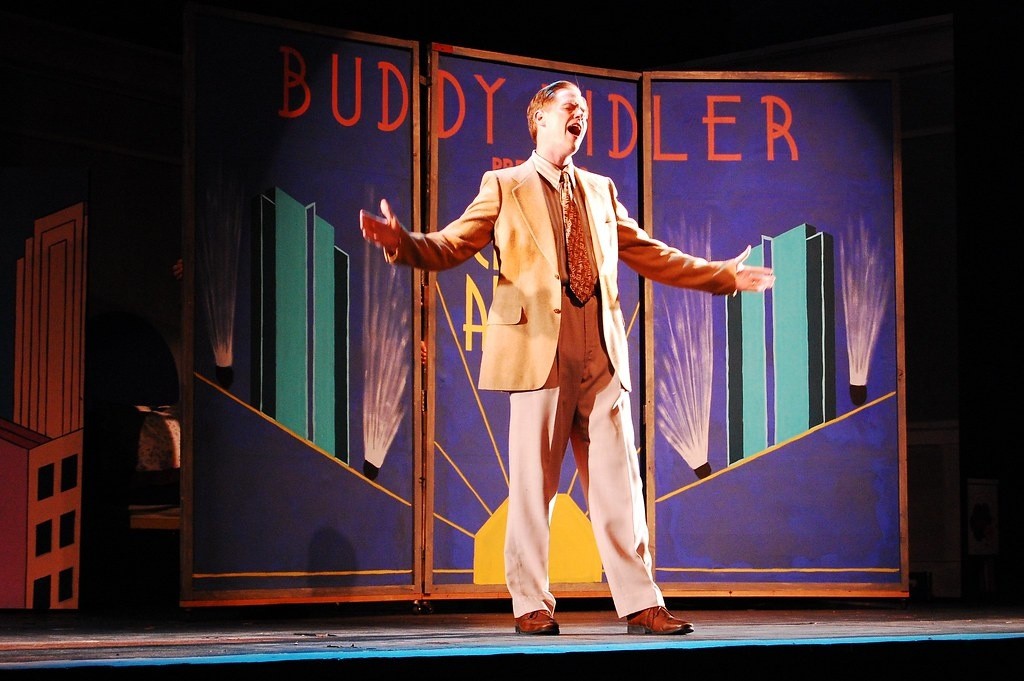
[752,281,755,285]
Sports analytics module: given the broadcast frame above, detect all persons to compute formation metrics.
[360,80,776,634]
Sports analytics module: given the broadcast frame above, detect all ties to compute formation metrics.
[560,171,594,304]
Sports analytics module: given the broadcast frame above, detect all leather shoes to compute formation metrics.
[514,610,560,635]
[627,606,695,635]
